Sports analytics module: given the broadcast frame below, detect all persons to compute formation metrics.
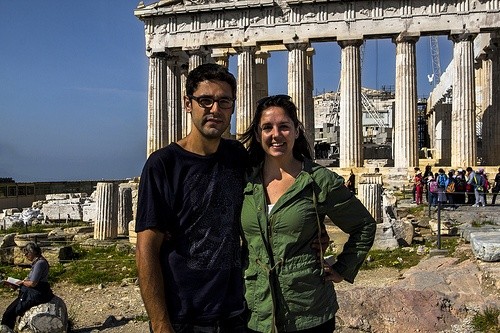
[135,62,253,333]
[314,141,500,209]
[0,242,53,333]
[237,94,397,333]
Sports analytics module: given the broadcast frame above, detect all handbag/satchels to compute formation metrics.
[476,184,483,191]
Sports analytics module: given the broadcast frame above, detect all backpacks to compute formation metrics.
[446,181,455,192]
[455,175,465,192]
[485,180,489,190]
[439,174,447,185]
[430,182,439,192]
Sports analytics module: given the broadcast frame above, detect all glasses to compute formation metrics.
[23,252,29,257]
[256,94,293,108]
[189,95,235,109]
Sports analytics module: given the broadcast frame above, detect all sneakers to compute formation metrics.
[482,204,485,207]
[472,204,479,207]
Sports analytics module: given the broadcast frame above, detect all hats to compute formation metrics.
[457,168,463,172]
[415,171,422,175]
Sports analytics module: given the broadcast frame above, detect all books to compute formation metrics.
[2,277,22,286]
